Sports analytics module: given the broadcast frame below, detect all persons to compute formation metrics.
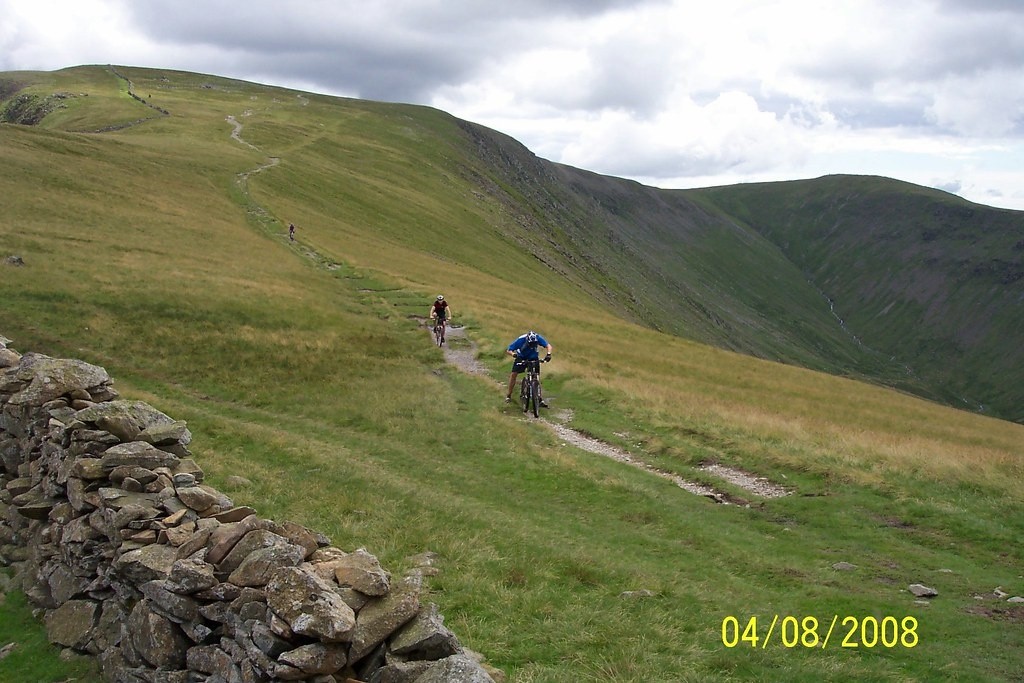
[290,223,295,237]
[430,295,452,343]
[505,330,552,408]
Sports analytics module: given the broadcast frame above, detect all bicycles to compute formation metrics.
[514,359,548,418]
[431,318,450,348]
[290,230,295,241]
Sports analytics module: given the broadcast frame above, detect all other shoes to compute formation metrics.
[442,338,445,342]
[434,329,436,333]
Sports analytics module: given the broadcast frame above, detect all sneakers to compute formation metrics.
[506,396,511,403]
[539,401,548,407]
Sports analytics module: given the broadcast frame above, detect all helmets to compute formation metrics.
[527,331,538,343]
[437,295,444,300]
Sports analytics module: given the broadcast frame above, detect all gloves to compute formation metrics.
[545,354,551,362]
[513,354,518,358]
